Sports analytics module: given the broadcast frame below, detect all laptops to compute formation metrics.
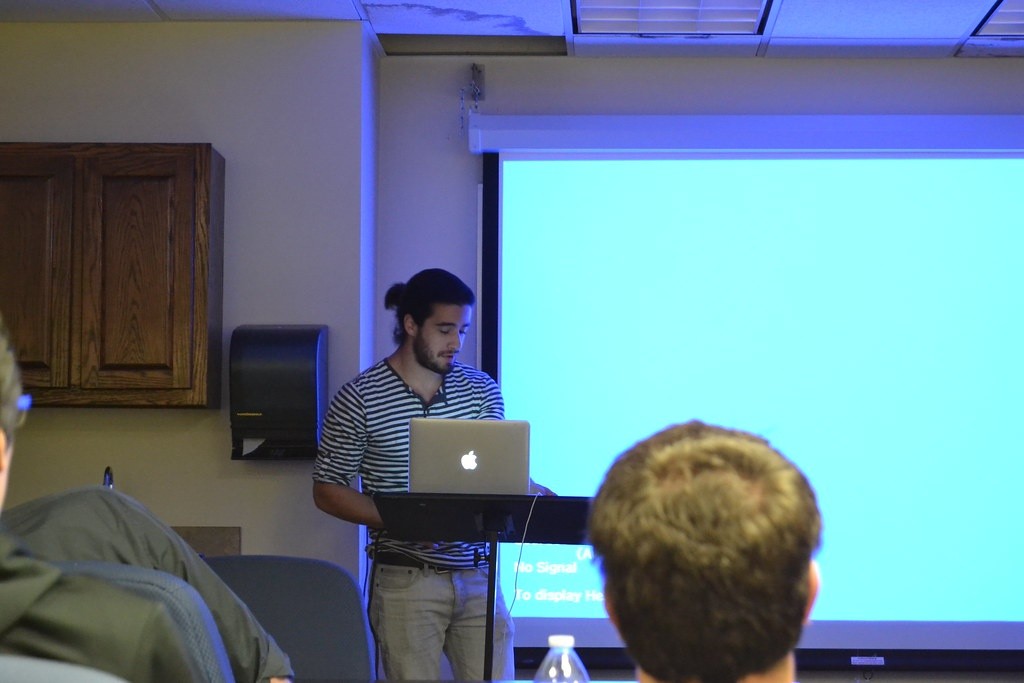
[407,417,530,494]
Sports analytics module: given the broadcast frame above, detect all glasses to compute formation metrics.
[13,396,32,428]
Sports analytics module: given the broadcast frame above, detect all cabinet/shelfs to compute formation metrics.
[0,142,226,407]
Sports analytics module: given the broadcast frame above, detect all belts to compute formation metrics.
[374,552,439,569]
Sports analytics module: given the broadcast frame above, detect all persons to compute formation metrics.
[588,419,825,683]
[312,269,557,682]
[0,337,294,683]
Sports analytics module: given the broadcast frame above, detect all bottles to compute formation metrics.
[534,635,590,683]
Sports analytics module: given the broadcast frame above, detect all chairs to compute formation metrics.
[203,554,378,683]
